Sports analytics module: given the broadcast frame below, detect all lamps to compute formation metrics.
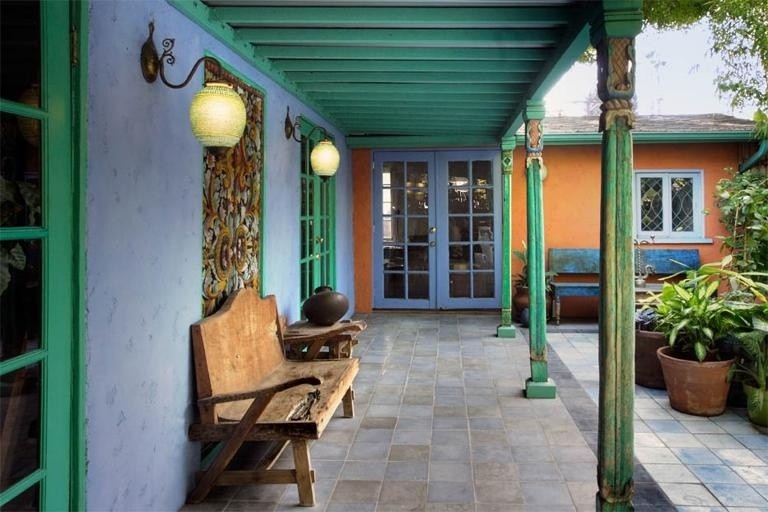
[137,16,249,153]
[281,103,344,186]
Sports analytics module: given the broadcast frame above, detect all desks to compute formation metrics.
[278,312,367,421]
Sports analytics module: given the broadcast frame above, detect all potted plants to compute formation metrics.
[508,236,559,327]
[633,249,768,438]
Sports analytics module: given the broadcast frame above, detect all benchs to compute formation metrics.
[542,242,702,326]
[189,284,363,509]
